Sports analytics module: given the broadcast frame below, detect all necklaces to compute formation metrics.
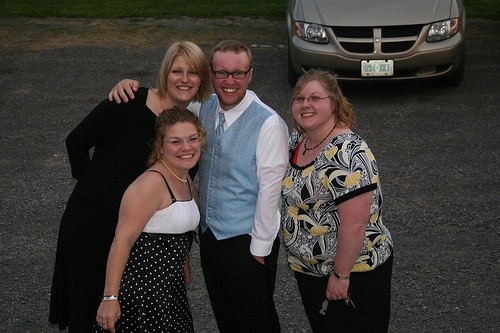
[303,123,336,154]
[160,159,188,183]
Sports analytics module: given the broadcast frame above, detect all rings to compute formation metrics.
[101,321,108,324]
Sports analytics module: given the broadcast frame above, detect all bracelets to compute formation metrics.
[102,295,118,301]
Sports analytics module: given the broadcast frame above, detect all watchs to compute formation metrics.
[333,270,350,280]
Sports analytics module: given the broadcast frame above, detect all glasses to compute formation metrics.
[292,95,330,105]
[212,66,252,80]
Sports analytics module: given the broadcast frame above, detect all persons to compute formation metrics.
[48,39,393,333]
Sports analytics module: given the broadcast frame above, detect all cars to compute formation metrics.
[285,0,467,89]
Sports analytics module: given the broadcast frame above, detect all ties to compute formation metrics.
[215,113,226,137]
